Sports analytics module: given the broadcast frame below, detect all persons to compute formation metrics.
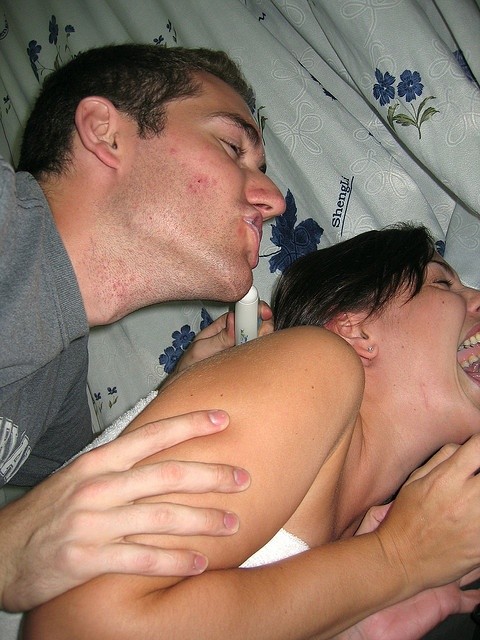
[0,221,480,640]
[0,44,286,614]
